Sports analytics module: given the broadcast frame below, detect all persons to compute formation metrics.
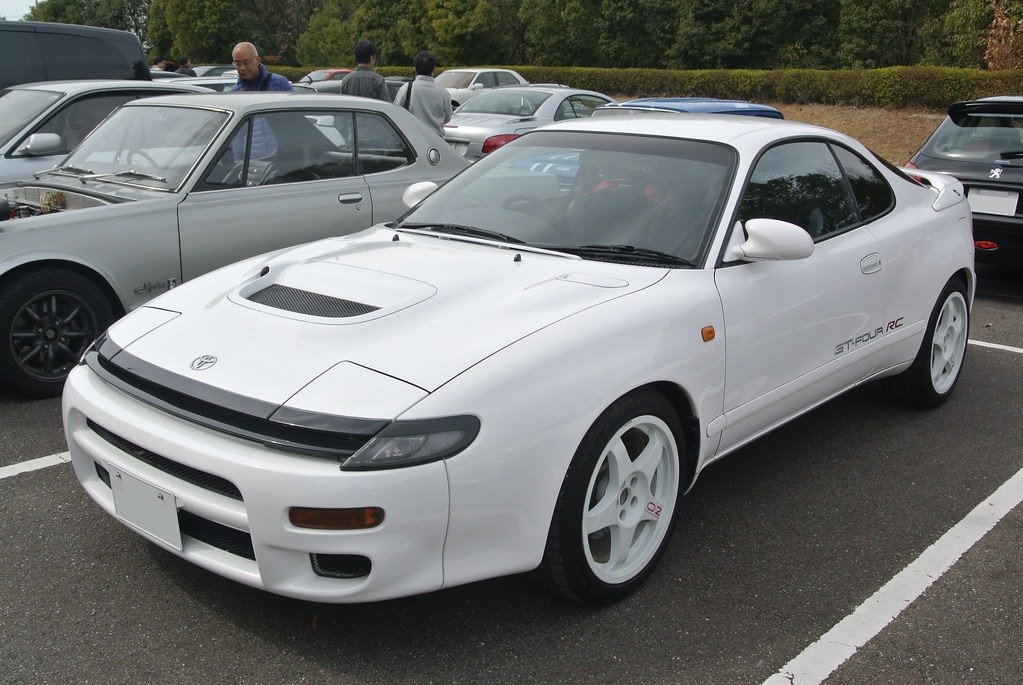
[164,58,179,72]
[339,42,391,151]
[392,50,452,149]
[149,55,166,71]
[174,57,197,78]
[230,41,294,161]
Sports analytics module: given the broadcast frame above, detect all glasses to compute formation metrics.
[232,57,256,68]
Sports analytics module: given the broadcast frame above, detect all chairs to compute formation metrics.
[222,159,273,186]
[990,125,1020,143]
[563,150,648,243]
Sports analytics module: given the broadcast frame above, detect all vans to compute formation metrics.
[1,19,151,92]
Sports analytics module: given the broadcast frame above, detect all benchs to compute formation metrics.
[315,151,409,179]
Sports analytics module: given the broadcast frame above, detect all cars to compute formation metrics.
[152,66,785,169]
[903,95,1023,299]
[1,80,222,188]
[0,93,564,399]
[62,113,979,602]
